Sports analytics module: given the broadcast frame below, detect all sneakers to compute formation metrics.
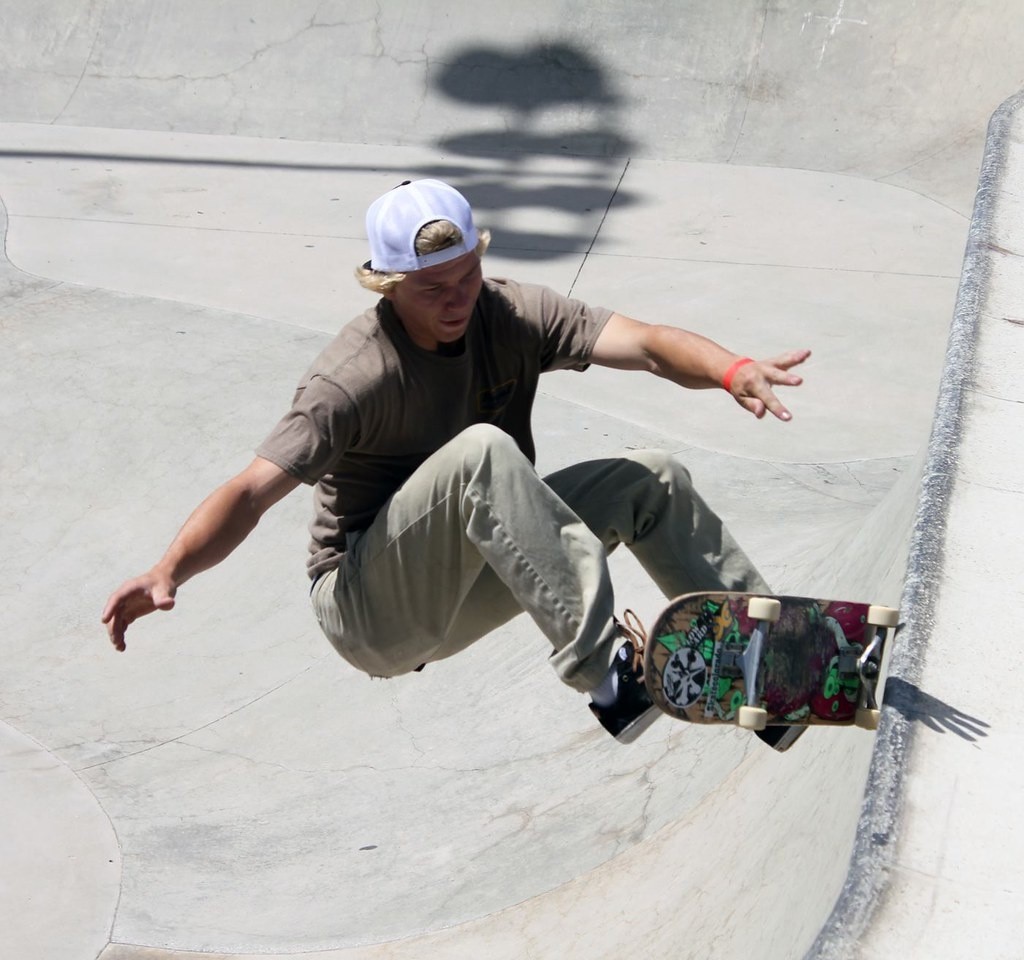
[750,724,811,755]
[587,607,663,747]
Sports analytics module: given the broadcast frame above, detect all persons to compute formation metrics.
[103,179,811,754]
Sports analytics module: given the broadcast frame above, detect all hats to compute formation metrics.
[357,175,481,275]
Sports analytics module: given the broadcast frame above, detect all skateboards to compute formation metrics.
[643,591,905,731]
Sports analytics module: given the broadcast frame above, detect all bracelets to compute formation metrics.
[723,358,754,391]
